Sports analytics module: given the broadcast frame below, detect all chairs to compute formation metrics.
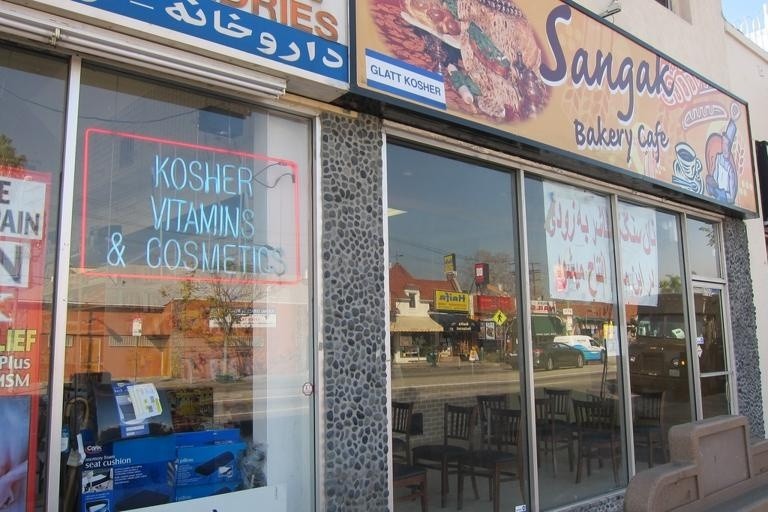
[391,381,669,512]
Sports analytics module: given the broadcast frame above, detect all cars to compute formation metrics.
[516,342,584,371]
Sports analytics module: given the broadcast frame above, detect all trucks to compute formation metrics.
[623,293,726,400]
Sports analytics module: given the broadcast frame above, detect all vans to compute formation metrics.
[554,336,606,365]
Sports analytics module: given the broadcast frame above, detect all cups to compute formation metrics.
[673,143,701,181]
